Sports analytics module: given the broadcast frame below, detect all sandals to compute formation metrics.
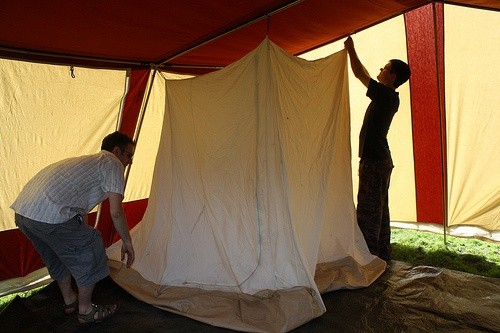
[77,302,120,325]
[63,296,78,315]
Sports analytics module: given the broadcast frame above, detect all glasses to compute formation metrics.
[384,66,391,73]
[123,149,134,159]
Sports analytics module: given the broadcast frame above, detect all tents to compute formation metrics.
[0,0,500,299]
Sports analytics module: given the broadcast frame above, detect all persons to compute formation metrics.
[9,131,135,326]
[344,35,411,266]
